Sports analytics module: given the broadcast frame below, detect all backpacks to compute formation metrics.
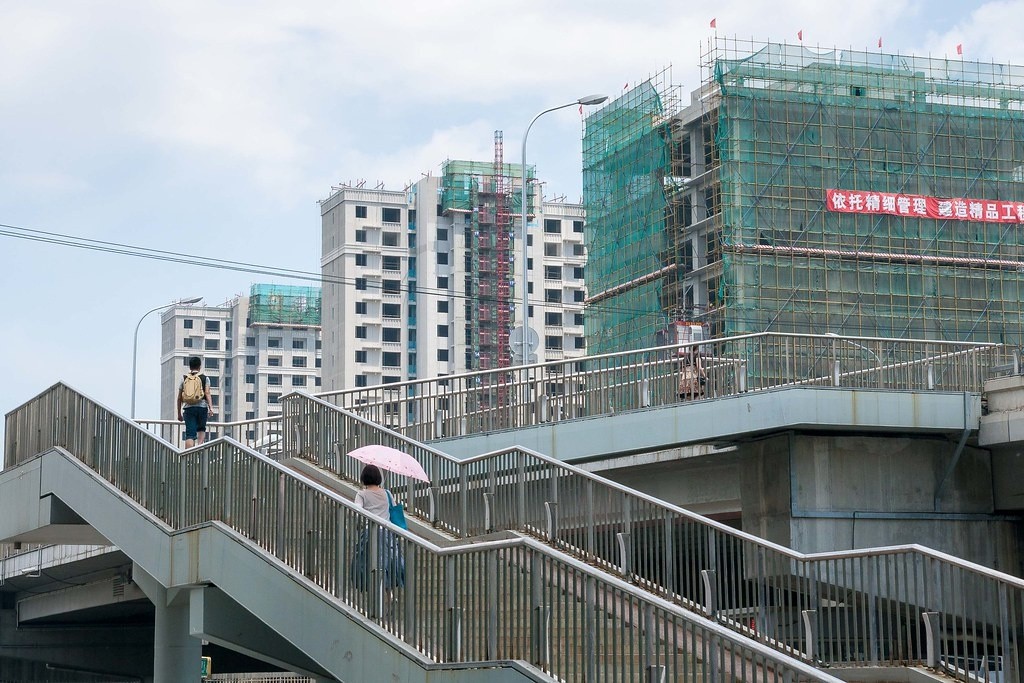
[181,372,205,404]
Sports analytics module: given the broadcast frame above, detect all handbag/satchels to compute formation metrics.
[697,376,709,385]
[385,489,408,530]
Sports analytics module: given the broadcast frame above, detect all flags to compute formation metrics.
[957,44,962,54]
[579,105,582,113]
[710,19,716,28]
[798,30,802,40]
[878,37,882,47]
[624,83,628,88]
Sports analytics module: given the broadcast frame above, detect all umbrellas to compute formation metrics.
[348,444,433,489]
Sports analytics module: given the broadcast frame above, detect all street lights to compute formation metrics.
[131,297,204,419]
[522,94,608,403]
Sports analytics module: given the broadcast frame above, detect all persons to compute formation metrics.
[176,355,215,463]
[353,465,398,618]
[678,343,709,401]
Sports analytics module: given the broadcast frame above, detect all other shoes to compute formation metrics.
[385,598,398,617]
